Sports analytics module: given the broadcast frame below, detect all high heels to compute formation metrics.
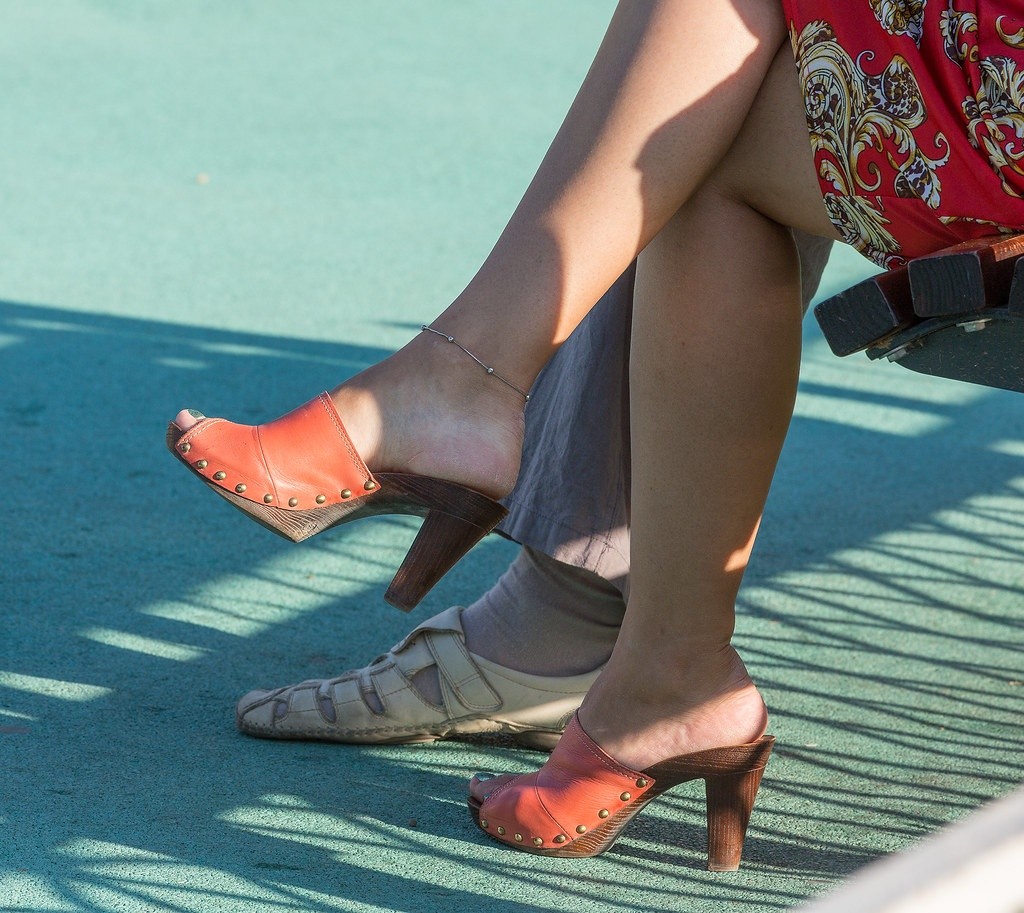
[467,708,776,873]
[167,391,509,613]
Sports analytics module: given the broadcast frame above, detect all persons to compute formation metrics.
[167,0,1024,872]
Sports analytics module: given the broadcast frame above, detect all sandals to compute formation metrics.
[236,604,605,753]
